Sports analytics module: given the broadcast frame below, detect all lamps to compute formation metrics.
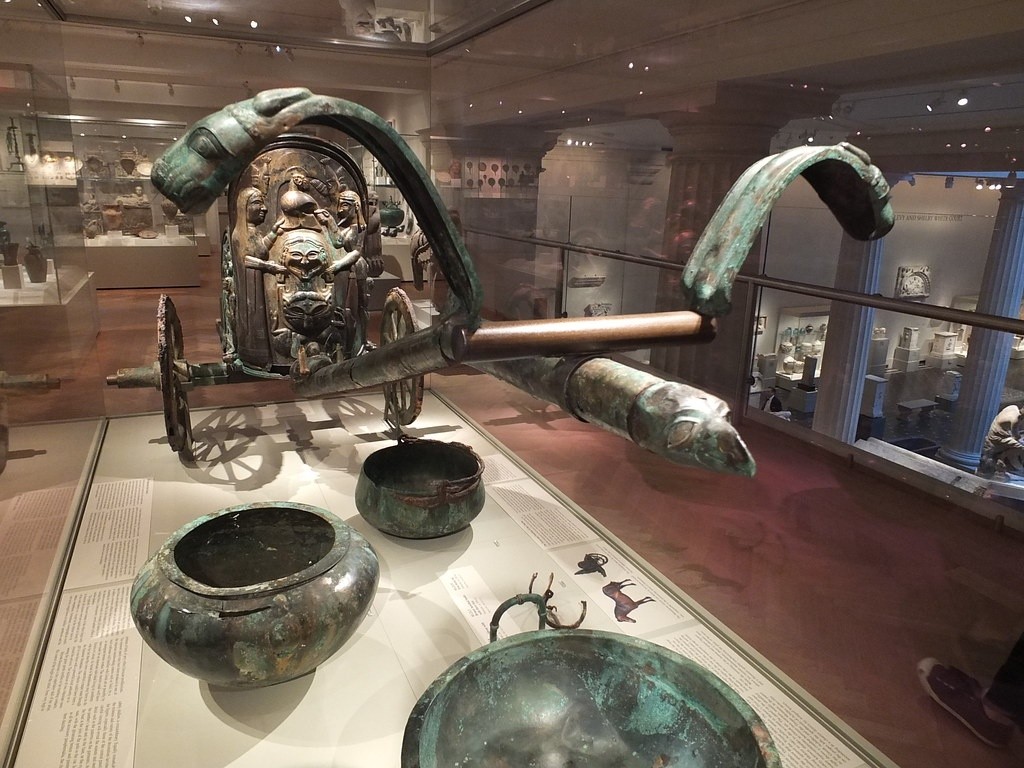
[68,75,175,99]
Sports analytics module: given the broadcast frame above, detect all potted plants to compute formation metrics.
[24,240,47,283]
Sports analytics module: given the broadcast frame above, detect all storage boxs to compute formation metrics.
[886,436,941,458]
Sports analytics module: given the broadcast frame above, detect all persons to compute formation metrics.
[124,186,148,203]
[984,404,1024,457]
[232,186,367,365]
[917,635,1024,746]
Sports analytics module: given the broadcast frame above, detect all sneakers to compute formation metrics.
[918,657,1015,748]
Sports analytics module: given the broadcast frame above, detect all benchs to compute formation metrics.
[894,397,940,422]
